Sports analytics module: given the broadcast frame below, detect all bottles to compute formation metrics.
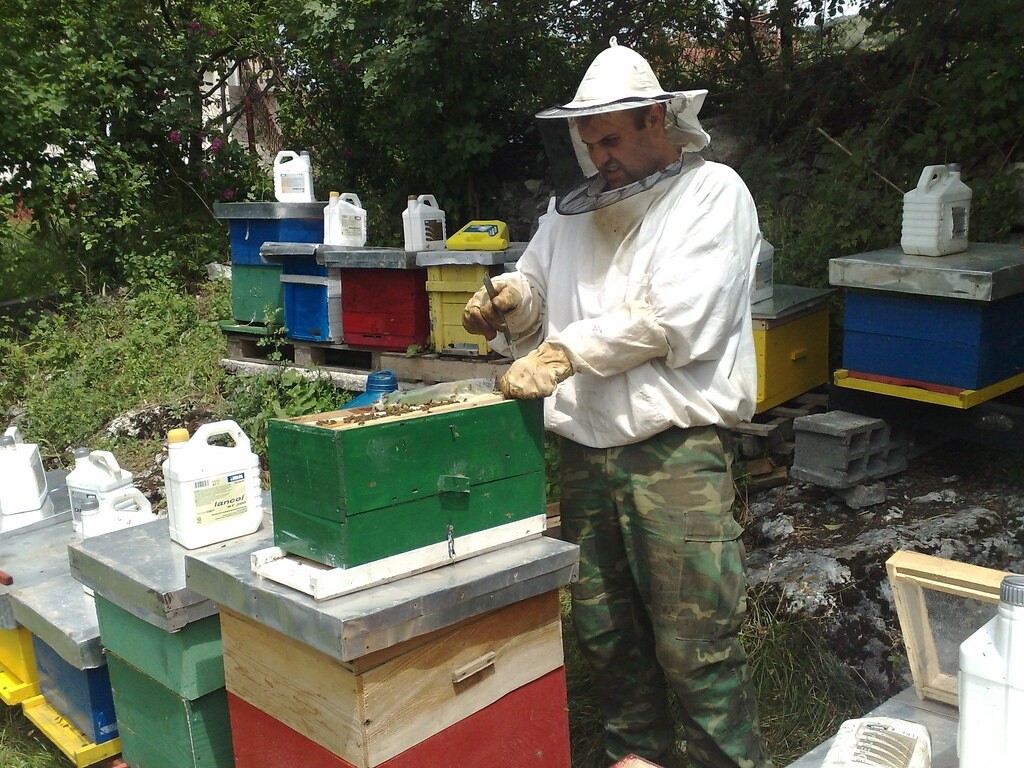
[900,162,972,257]
[446,220,509,251]
[322,191,367,246]
[957,573,1024,767]
[338,370,399,411]
[819,716,933,768]
[65,446,134,533]
[273,149,315,203]
[79,486,157,598]
[163,419,263,550]
[750,232,774,304]
[402,194,447,251]
[0,425,48,515]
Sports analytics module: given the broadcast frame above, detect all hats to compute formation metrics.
[534,35,709,214]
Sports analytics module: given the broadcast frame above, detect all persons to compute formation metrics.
[462,38,767,768]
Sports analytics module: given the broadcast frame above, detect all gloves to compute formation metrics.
[461,273,542,341]
[500,299,669,401]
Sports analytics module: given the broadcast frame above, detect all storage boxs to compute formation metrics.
[265,384,554,569]
[93,591,226,701]
[843,286,1024,390]
[227,665,572,768]
[229,218,324,266]
[753,302,829,414]
[217,588,564,768]
[231,264,284,324]
[341,268,429,352]
[103,648,235,768]
[0,627,38,685]
[32,632,119,745]
